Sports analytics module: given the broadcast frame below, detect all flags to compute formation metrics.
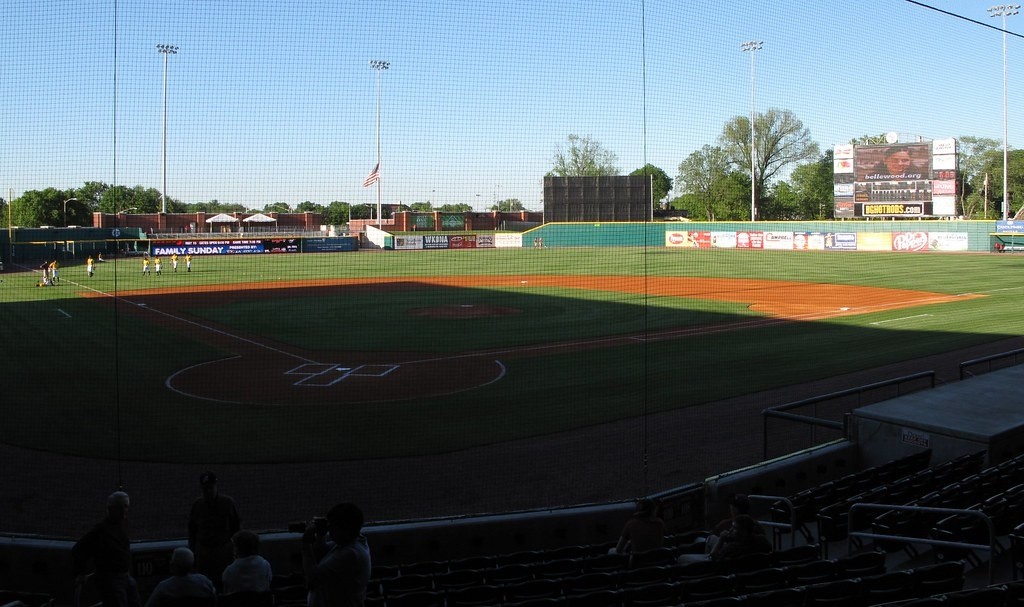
[363,163,379,187]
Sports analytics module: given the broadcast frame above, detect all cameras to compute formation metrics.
[286,517,328,536]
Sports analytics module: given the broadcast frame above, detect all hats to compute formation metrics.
[634,499,656,515]
[200,472,217,489]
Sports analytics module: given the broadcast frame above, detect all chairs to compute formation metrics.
[0,438,1024,607]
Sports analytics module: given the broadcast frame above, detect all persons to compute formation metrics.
[170,253,179,272]
[183,253,192,272]
[222,530,273,596]
[121,247,151,257]
[99,252,105,262]
[300,503,371,607]
[154,256,163,276]
[142,257,150,276]
[534,237,543,250]
[615,499,665,561]
[73,491,142,607]
[87,255,96,276]
[184,224,190,233]
[994,243,1004,252]
[144,547,217,607]
[34,259,61,287]
[865,146,927,180]
[694,493,773,564]
[189,471,241,594]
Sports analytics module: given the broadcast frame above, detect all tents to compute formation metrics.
[206,214,240,233]
[243,213,277,232]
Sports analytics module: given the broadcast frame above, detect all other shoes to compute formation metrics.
[142,275,151,277]
[156,274,161,276]
